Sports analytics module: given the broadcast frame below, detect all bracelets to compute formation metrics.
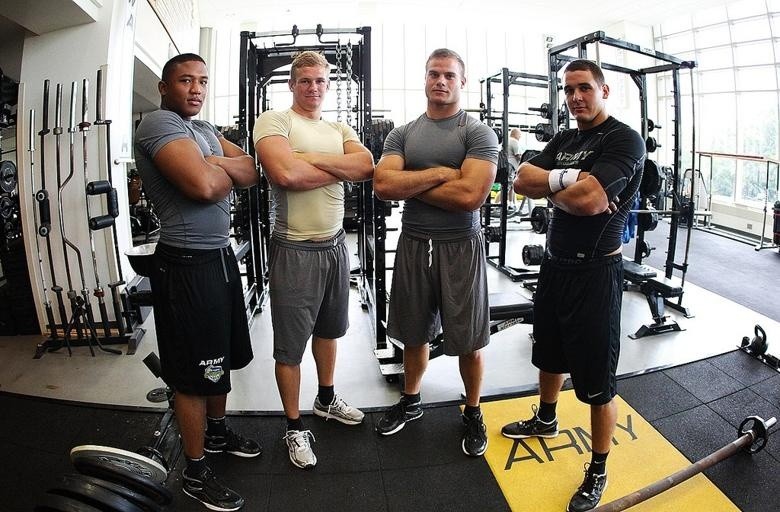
[549,168,581,193]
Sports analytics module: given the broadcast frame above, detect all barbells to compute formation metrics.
[521,203,712,235]
[585,415,776,510]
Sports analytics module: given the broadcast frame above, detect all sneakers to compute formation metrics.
[490,205,516,214]
[501,409,560,439]
[181,467,245,512]
[313,394,365,425]
[461,410,489,457]
[566,465,607,512]
[375,397,423,436]
[285,424,317,472]
[203,428,263,458]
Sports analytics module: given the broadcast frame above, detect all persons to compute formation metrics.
[251,51,375,472]
[134,53,263,512]
[494,129,523,209]
[372,48,500,457]
[502,60,647,512]
